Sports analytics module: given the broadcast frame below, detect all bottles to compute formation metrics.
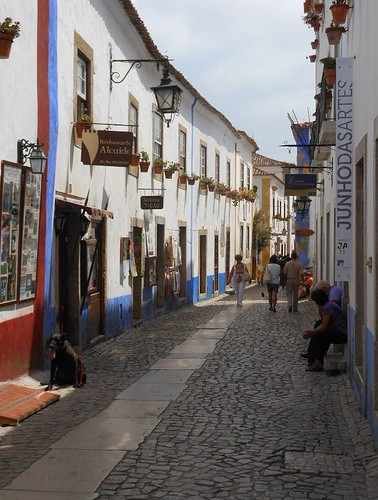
[261,293,265,297]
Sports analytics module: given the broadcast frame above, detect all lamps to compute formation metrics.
[17,139,49,173]
[110,42,182,128]
[294,196,312,221]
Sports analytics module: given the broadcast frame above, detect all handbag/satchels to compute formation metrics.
[243,264,250,282]
[263,265,271,285]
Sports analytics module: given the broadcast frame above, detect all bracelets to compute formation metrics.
[314,329,318,334]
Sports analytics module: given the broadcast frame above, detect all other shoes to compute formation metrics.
[294,309,298,312]
[269,307,276,312]
[306,361,323,371]
[301,353,309,358]
[289,305,292,312]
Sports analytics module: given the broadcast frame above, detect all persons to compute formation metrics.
[302,288,348,371]
[300,280,342,358]
[227,254,251,307]
[265,255,281,313]
[278,254,314,312]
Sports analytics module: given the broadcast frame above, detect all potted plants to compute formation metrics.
[200,176,257,206]
[0,17,20,56]
[302,0,349,114]
[188,174,195,185]
[154,157,163,173]
[165,161,178,178]
[179,172,187,183]
[140,151,150,172]
[76,113,91,138]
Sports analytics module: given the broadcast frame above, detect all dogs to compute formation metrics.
[40,332,87,391]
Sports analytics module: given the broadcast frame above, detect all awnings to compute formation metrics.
[55,194,114,219]
